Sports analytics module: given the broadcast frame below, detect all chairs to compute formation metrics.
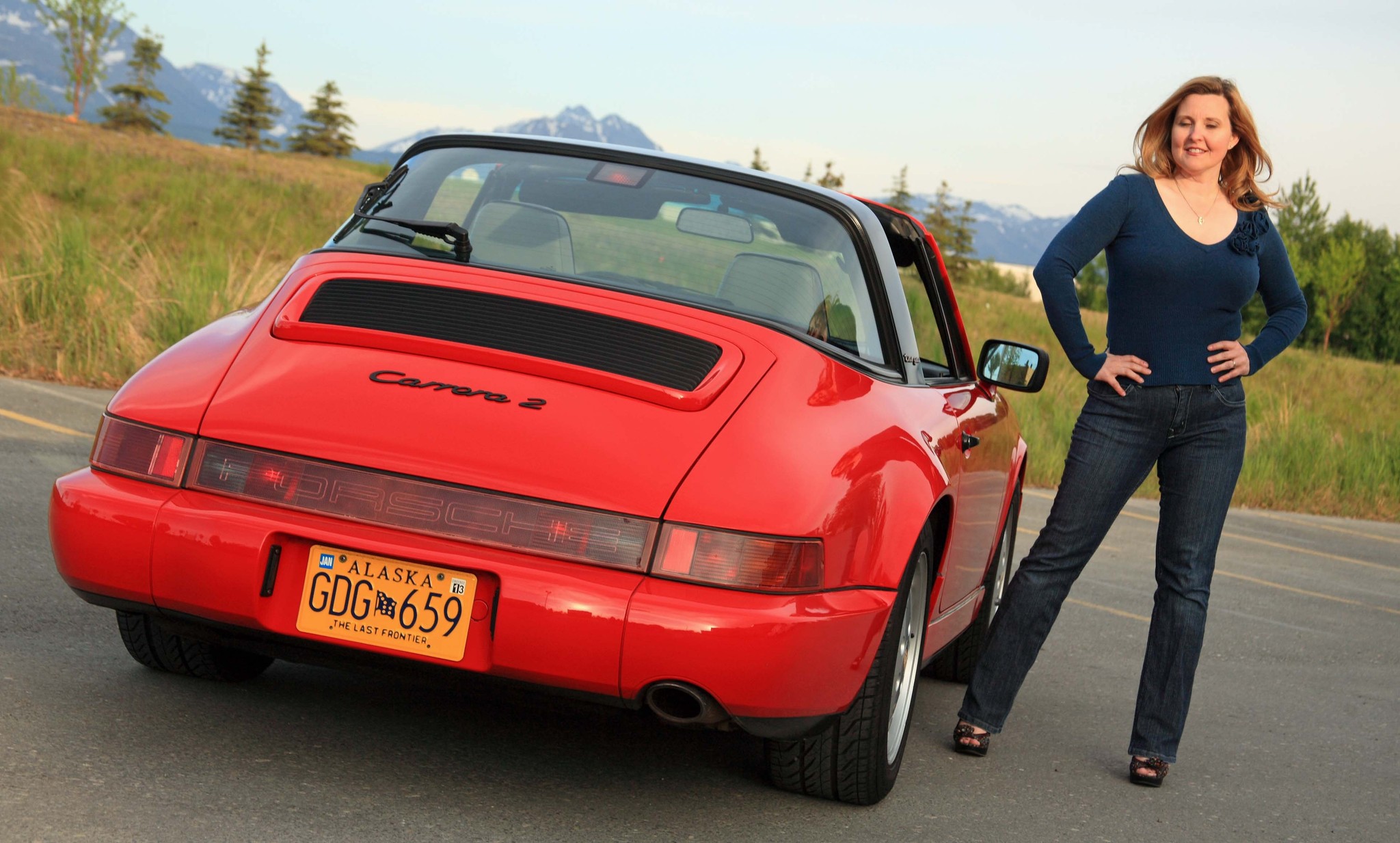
[470,198,576,275]
[717,253,830,342]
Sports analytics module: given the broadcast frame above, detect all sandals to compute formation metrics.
[953,719,990,756]
[1129,754,1168,787]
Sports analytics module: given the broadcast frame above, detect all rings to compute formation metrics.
[1232,359,1236,369]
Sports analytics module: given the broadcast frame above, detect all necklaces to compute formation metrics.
[1172,179,1220,225]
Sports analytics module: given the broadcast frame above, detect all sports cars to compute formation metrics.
[43,127,1053,810]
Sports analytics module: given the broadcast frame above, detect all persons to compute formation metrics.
[954,76,1309,787]
